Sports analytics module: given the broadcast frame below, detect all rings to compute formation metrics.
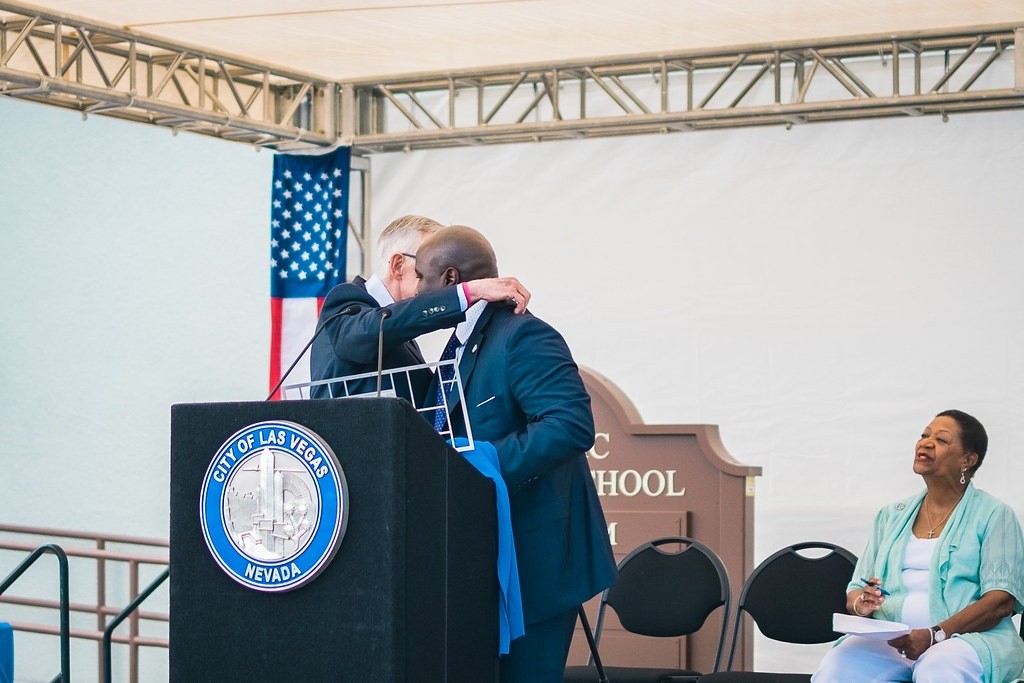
[859,594,866,601]
[901,651,907,659]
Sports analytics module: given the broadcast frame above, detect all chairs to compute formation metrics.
[696,541,874,683]
[563,535,733,683]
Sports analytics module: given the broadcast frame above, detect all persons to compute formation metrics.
[309,215,533,420]
[809,410,1024,683]
[416,224,616,683]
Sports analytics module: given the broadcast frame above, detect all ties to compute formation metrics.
[434,327,461,432]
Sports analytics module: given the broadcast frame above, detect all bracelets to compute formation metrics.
[853,598,871,617]
[927,627,934,648]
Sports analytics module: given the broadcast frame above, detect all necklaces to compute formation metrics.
[925,493,964,537]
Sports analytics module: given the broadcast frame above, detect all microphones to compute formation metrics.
[267,305,361,401]
[376,309,393,398]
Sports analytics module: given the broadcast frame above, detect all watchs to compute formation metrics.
[931,624,946,642]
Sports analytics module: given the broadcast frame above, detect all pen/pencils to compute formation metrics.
[860,577,891,596]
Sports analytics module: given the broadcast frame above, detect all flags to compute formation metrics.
[269,135,353,400]
